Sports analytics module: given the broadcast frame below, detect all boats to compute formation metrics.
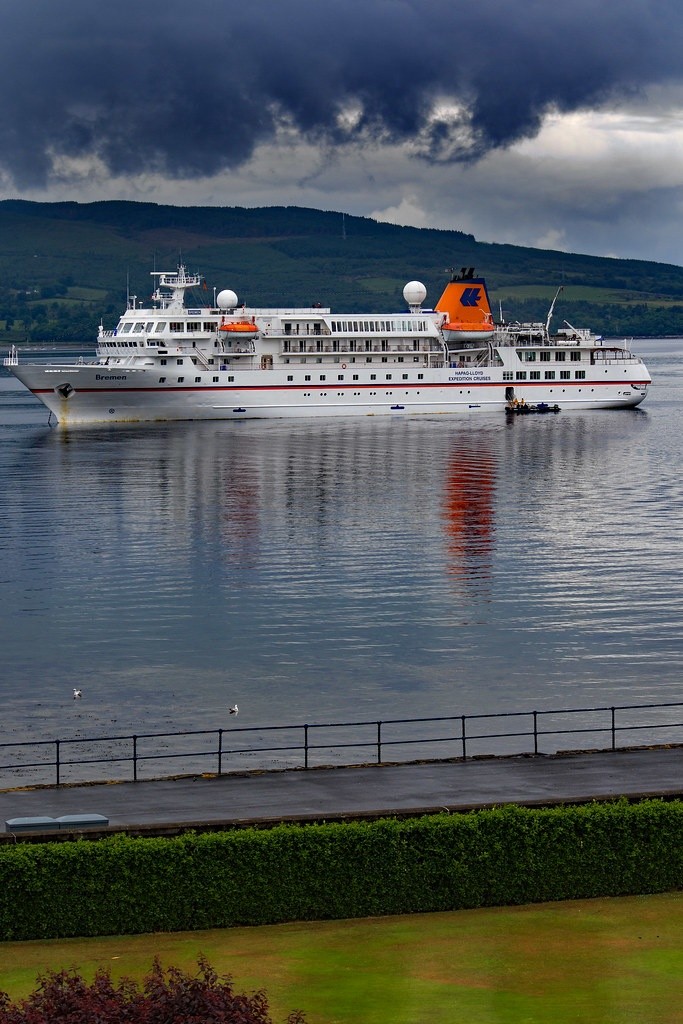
[218,323,258,338]
[504,401,561,414]
[3,249,652,423]
[442,322,495,341]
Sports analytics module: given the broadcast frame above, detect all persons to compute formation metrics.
[316,303,320,307]
[521,398,525,406]
[453,362,462,368]
[514,398,518,405]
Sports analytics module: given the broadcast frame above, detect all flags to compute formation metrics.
[203,280,207,290]
